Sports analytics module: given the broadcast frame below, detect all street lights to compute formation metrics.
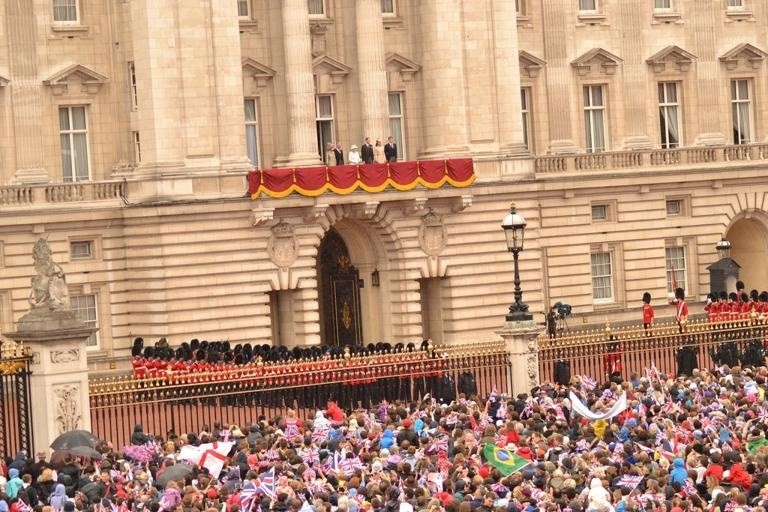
[495,203,546,326]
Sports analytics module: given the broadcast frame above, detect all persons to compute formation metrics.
[373,139,384,162]
[348,145,361,163]
[361,137,373,164]
[384,136,397,162]
[642,282,768,335]
[325,143,344,166]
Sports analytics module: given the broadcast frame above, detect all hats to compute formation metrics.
[708,279,768,302]
[675,288,684,300]
[642,292,651,304]
[131,337,431,363]
